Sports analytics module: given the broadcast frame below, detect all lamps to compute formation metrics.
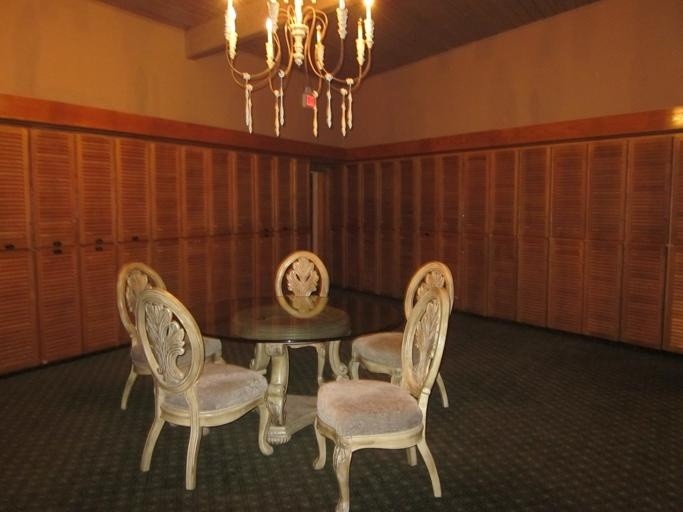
[222,0,375,140]
